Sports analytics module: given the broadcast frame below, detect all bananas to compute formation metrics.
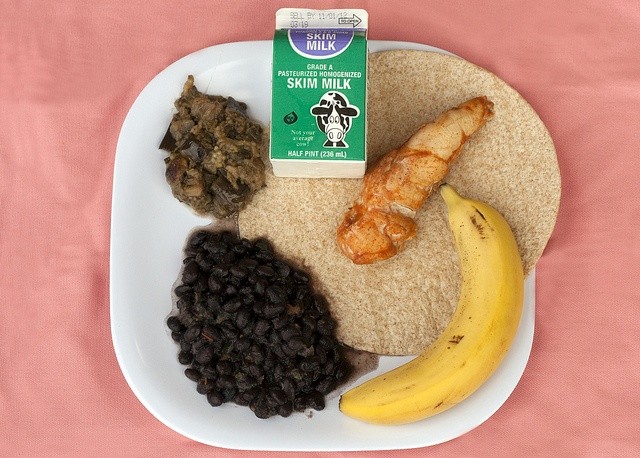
[339,182,524,426]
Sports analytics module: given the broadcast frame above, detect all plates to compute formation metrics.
[110,40,537,451]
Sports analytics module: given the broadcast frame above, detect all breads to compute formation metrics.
[336,96,494,265]
[237,47,563,355]
